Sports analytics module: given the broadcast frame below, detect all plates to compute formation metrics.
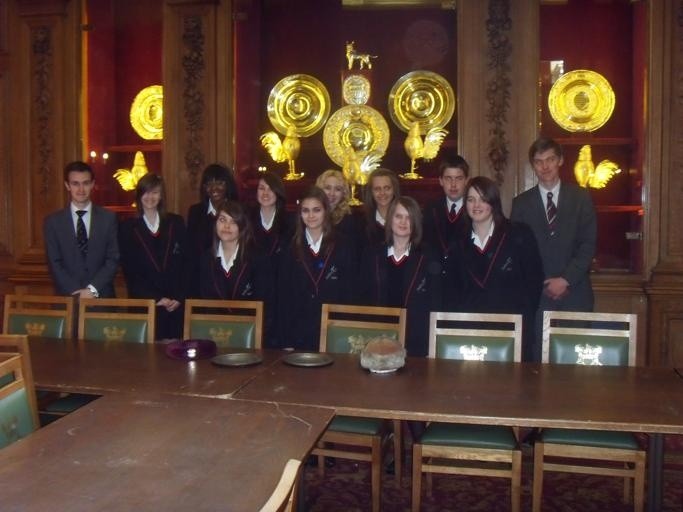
[209,352,265,368]
[370,368,398,373]
[283,352,335,369]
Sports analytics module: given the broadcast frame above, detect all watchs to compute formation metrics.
[87,286,100,298]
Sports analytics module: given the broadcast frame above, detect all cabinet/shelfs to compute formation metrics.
[90,142,162,216]
[543,135,643,215]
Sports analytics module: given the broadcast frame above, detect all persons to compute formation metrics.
[313,169,365,241]
[423,154,476,258]
[273,185,354,468]
[361,167,400,264]
[358,196,442,473]
[196,201,257,313]
[446,176,544,361]
[509,138,596,448]
[42,162,124,338]
[245,171,294,350]
[115,172,183,339]
[186,164,236,269]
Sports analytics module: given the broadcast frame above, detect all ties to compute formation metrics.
[546,192,556,222]
[75,210,88,262]
[449,203,458,221]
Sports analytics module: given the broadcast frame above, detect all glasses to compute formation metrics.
[201,181,226,187]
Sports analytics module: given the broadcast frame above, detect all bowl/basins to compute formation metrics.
[165,339,219,361]
